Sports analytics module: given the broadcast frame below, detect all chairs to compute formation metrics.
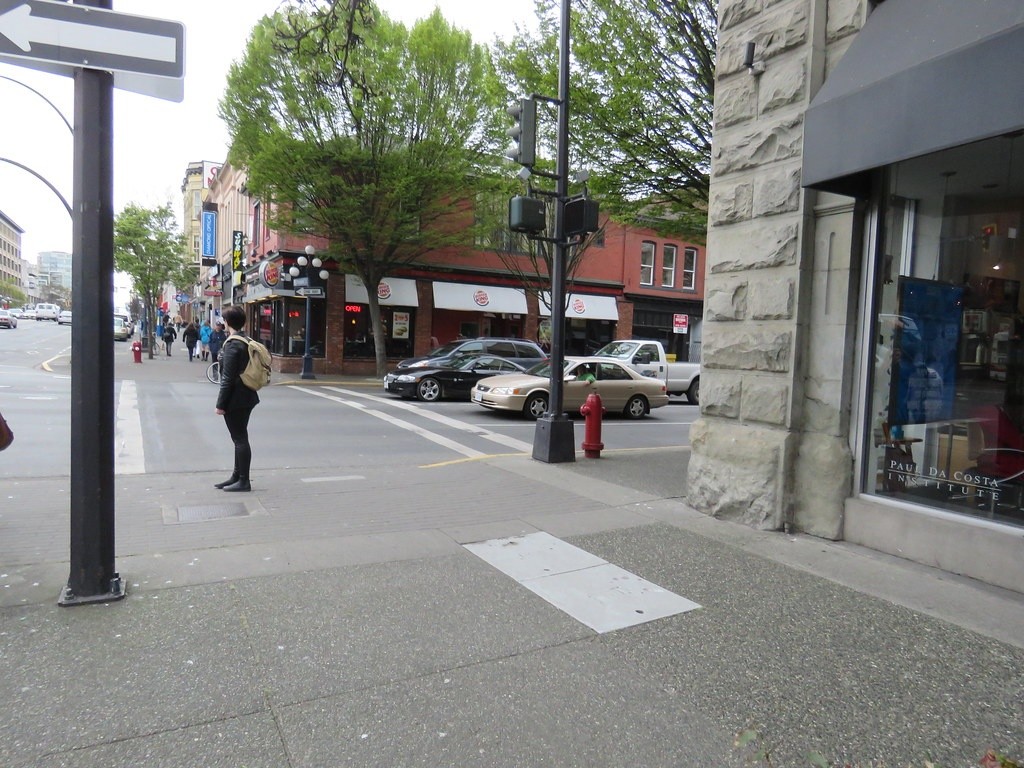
[964,422,1024,519]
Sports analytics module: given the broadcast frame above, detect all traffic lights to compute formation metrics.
[564,197,599,237]
[504,97,536,168]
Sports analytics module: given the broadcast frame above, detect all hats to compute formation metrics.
[216,321,221,326]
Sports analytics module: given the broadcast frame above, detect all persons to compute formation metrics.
[576,364,596,386]
[209,321,227,385]
[192,325,200,358]
[193,319,201,330]
[162,322,177,356]
[199,321,212,361]
[174,311,183,332]
[216,306,259,492]
[183,323,199,361]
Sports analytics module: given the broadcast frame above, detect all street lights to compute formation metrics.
[287,243,331,381]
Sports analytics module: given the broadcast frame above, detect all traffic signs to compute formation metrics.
[295,287,323,297]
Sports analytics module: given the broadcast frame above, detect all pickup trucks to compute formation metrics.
[592,340,700,407]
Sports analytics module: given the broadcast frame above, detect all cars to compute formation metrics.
[469,356,670,420]
[381,334,552,402]
[114,312,135,342]
[57,310,73,325]
[0,302,61,329]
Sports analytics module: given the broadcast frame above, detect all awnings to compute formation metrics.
[432,281,529,316]
[345,273,419,308]
[801,0,1024,202]
[538,289,621,321]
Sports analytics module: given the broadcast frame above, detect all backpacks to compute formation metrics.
[222,335,272,391]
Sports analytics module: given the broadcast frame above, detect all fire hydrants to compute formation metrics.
[580,394,609,460]
[130,340,142,363]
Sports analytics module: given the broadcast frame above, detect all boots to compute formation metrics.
[215,469,240,488]
[222,473,251,492]
[201,351,205,361]
[205,352,209,361]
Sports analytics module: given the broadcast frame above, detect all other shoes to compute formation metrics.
[197,354,200,358]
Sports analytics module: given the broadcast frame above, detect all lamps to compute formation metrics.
[246,250,272,268]
[743,42,765,76]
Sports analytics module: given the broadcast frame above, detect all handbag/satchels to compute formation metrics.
[163,330,171,339]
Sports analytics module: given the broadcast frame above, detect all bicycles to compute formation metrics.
[206,361,220,384]
